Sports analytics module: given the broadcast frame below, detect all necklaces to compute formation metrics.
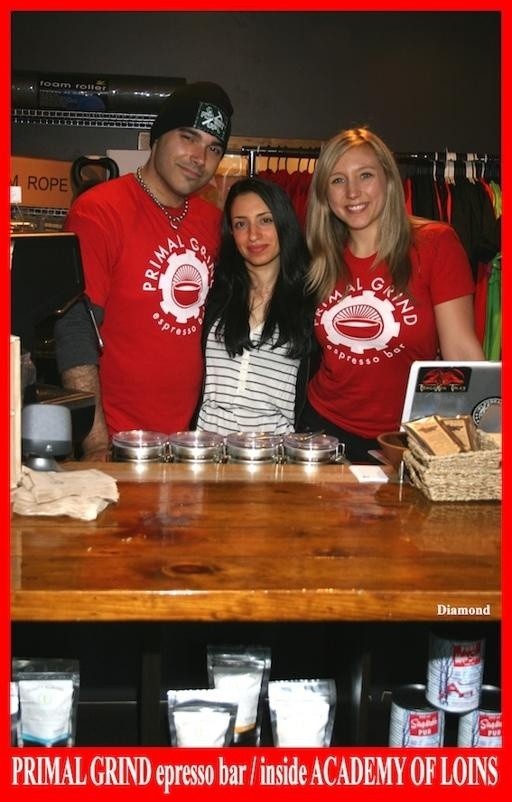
[136,167,189,230]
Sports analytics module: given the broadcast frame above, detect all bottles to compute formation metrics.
[112,430,343,464]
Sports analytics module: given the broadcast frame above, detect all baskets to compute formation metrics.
[407,428,503,468]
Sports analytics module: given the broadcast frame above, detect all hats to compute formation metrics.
[148,81,234,156]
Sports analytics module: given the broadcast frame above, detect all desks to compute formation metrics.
[10,460,501,749]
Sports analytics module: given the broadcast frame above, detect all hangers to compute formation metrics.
[255,143,490,187]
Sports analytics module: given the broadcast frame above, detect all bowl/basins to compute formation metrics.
[378,433,406,465]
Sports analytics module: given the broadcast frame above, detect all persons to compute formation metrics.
[51,80,234,464]
[189,177,320,447]
[303,128,488,466]
